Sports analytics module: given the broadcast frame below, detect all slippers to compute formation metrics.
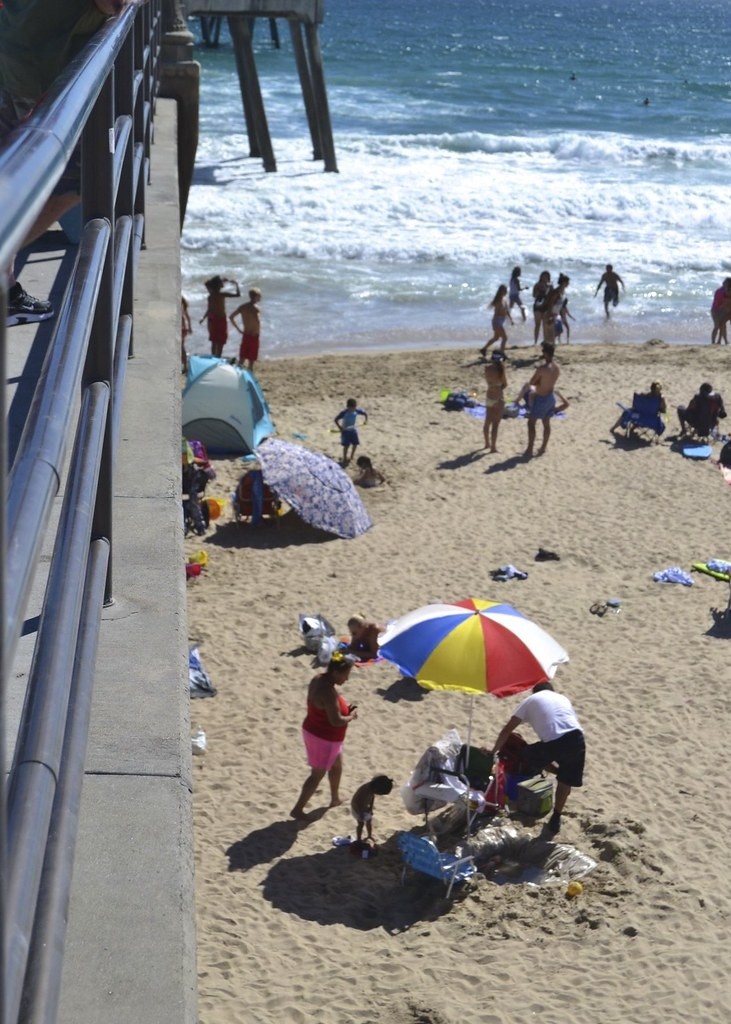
[591,602,608,615]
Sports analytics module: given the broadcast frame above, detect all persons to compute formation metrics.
[351,775,393,839]
[481,682,586,832]
[199,276,240,356]
[181,295,193,374]
[611,381,727,439]
[289,651,358,819]
[0,0,124,328]
[229,288,261,370]
[346,610,386,660]
[594,264,625,319]
[335,399,368,465]
[483,350,507,452]
[515,341,569,455]
[354,456,386,489]
[710,278,731,344]
[477,266,576,357]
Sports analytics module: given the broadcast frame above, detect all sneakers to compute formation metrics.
[5,284,55,329]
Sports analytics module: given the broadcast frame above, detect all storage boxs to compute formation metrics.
[516,779,552,815]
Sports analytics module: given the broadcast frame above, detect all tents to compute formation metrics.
[182,354,275,454]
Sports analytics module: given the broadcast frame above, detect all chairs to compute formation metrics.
[397,831,478,899]
[615,401,660,442]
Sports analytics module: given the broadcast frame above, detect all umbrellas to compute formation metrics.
[252,435,372,539]
[376,597,570,771]
